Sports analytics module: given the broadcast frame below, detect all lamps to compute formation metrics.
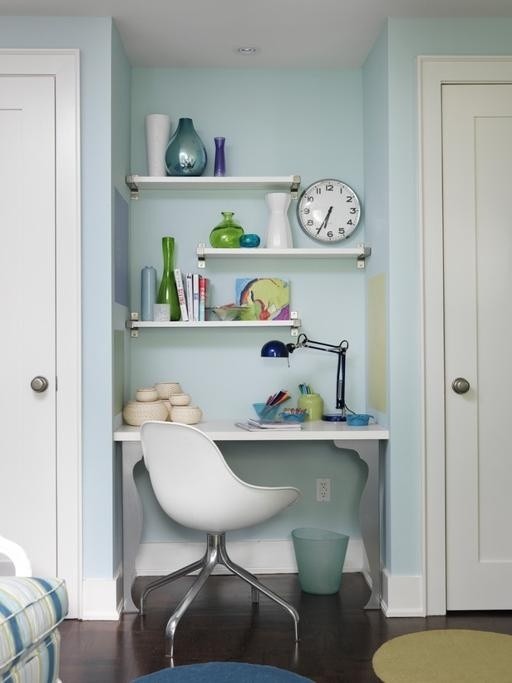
[261,333,349,421]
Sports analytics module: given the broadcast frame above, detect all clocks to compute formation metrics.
[297,178,361,243]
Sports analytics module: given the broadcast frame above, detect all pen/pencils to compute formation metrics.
[284,408,306,414]
[299,382,314,394]
[266,390,291,405]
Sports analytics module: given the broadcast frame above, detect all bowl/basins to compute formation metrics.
[346,414,370,426]
[206,306,250,322]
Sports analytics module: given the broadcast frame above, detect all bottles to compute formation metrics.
[297,393,324,420]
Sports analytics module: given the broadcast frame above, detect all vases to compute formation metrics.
[264,193,294,248]
[210,211,244,248]
[144,114,226,176]
[158,236,181,320]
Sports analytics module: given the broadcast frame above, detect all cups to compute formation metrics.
[253,403,279,422]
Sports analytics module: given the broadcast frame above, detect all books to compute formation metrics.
[236,422,302,432]
[186,273,193,321]
[173,268,188,322]
[199,278,206,321]
[247,417,302,429]
[192,273,199,321]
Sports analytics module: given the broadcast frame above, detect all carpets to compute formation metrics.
[372,627,512,682]
[132,662,314,683]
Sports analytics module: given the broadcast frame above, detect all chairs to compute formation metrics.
[140,418,301,658]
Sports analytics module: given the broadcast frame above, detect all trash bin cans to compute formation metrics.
[291,527,349,595]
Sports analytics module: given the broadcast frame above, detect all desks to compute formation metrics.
[113,423,389,612]
